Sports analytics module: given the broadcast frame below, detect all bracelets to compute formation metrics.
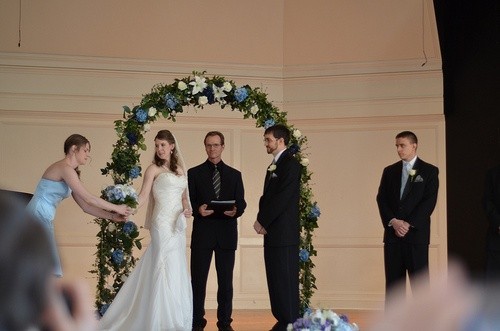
[112,213,114,220]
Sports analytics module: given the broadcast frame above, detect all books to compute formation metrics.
[205,200,236,218]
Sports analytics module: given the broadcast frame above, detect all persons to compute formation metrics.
[130,130,193,331]
[188,131,247,331]
[254,124,302,331]
[376,131,439,316]
[27,134,132,280]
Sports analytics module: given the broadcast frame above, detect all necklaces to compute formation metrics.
[162,165,173,173]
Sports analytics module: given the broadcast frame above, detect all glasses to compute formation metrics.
[206,144,222,149]
[265,138,279,144]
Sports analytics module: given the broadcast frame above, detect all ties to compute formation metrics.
[212,165,221,200]
[269,159,275,179]
[400,164,407,200]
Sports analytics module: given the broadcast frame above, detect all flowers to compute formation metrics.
[104,184,140,218]
[287,308,359,331]
[89,71,321,321]
[267,163,277,179]
[409,170,417,182]
